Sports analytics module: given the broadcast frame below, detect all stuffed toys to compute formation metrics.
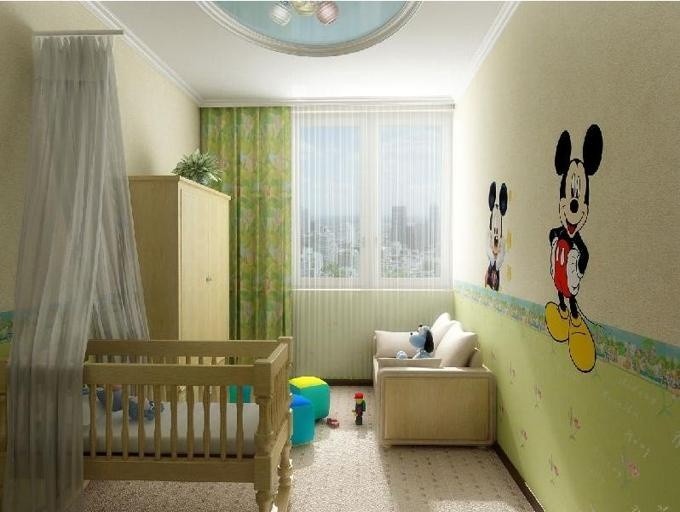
[396,324,434,359]
[96,384,165,422]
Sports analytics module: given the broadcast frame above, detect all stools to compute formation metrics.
[227,376,330,446]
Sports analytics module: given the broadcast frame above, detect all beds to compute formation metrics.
[0,335,295,511]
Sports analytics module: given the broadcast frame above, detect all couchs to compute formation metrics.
[371,312,497,451]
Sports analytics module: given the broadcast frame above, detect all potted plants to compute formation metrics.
[172,148,226,186]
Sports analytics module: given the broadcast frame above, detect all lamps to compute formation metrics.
[267,1,341,27]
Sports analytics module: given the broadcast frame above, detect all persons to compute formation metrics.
[352,392,366,425]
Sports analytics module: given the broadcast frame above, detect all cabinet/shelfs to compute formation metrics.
[128,175,232,403]
